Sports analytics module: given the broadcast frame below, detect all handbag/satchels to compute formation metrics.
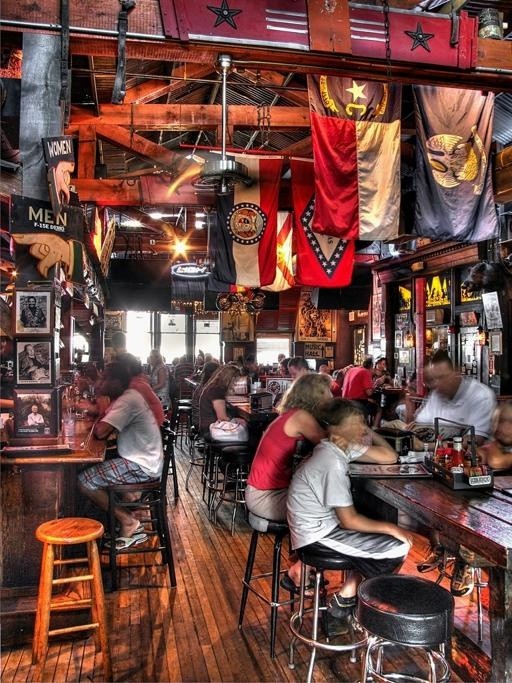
[208,419,249,442]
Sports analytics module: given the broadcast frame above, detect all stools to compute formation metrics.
[33,514,110,668]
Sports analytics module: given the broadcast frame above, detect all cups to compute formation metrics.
[251,383,258,394]
[62,419,75,435]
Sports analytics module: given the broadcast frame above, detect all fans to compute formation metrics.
[186,53,272,197]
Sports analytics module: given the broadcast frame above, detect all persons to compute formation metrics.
[77,330,171,550]
[20,296,44,329]
[46,158,76,213]
[300,306,328,338]
[19,342,50,380]
[169,349,512,639]
[0,337,16,415]
[24,404,45,429]
[10,230,88,288]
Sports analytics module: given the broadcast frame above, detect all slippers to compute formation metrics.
[103,525,144,539]
[283,568,329,585]
[280,576,328,599]
[104,534,148,551]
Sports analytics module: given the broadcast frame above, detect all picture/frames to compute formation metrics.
[12,387,59,440]
[488,331,504,356]
[11,286,54,338]
[12,338,54,388]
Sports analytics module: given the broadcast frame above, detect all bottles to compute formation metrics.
[394,373,400,387]
[424,436,483,470]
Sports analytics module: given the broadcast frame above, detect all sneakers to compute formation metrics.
[327,592,357,619]
[416,544,444,573]
[450,544,474,597]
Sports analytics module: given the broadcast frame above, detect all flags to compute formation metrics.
[205,275,253,294]
[257,208,296,292]
[304,73,405,244]
[288,155,357,290]
[206,148,282,287]
[409,82,505,245]
